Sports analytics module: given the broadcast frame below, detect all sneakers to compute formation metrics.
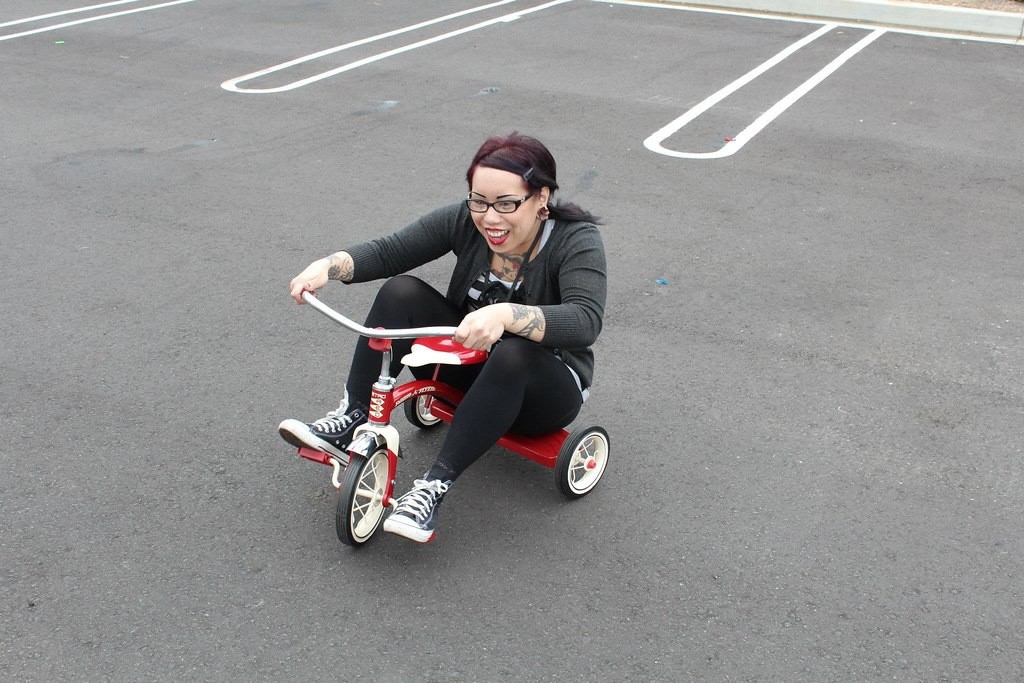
[383,482,453,542]
[278,409,367,467]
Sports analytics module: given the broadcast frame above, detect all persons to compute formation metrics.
[290,131,608,544]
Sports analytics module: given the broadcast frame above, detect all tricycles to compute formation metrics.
[295,288,611,550]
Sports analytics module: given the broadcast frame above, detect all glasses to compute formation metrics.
[465,191,534,213]
